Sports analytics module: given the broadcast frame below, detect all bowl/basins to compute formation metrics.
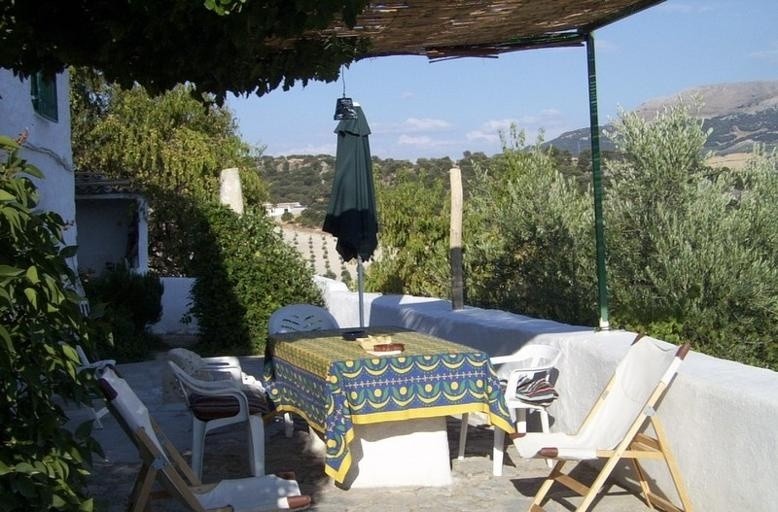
[340,330,364,342]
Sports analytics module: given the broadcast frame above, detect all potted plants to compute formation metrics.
[96,258,166,361]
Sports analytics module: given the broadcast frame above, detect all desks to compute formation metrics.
[264,326,487,493]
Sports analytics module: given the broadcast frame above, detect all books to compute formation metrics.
[355,335,401,356]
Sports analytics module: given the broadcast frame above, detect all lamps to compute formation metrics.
[333,52,356,119]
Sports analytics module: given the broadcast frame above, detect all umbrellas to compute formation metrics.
[320,99,380,334]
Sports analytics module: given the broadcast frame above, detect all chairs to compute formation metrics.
[517,331,694,511]
[266,304,340,334]
[458,342,563,480]
[97,362,313,511]
[166,344,276,486]
